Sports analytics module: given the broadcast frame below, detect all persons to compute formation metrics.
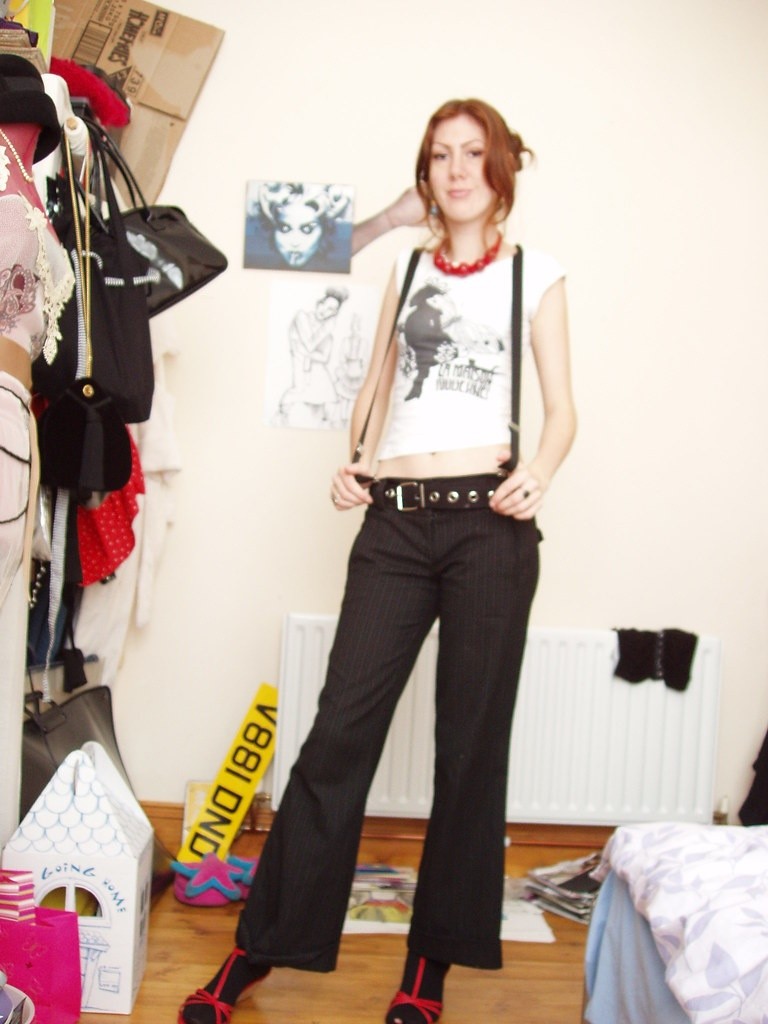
[179,99,580,1023]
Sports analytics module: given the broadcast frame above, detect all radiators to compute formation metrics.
[272,612,721,828]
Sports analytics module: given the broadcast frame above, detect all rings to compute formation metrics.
[521,485,529,499]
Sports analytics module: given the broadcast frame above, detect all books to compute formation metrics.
[527,848,603,927]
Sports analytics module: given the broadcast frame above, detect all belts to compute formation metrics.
[370,477,507,509]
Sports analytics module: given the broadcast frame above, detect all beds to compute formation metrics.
[582,822,768,1024]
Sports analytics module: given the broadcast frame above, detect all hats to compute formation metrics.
[0,54,61,164]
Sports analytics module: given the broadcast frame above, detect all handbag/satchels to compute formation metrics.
[20,686,181,913]
[48,117,228,422]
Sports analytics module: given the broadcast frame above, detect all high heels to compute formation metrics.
[383,957,444,1024]
[178,945,272,1024]
